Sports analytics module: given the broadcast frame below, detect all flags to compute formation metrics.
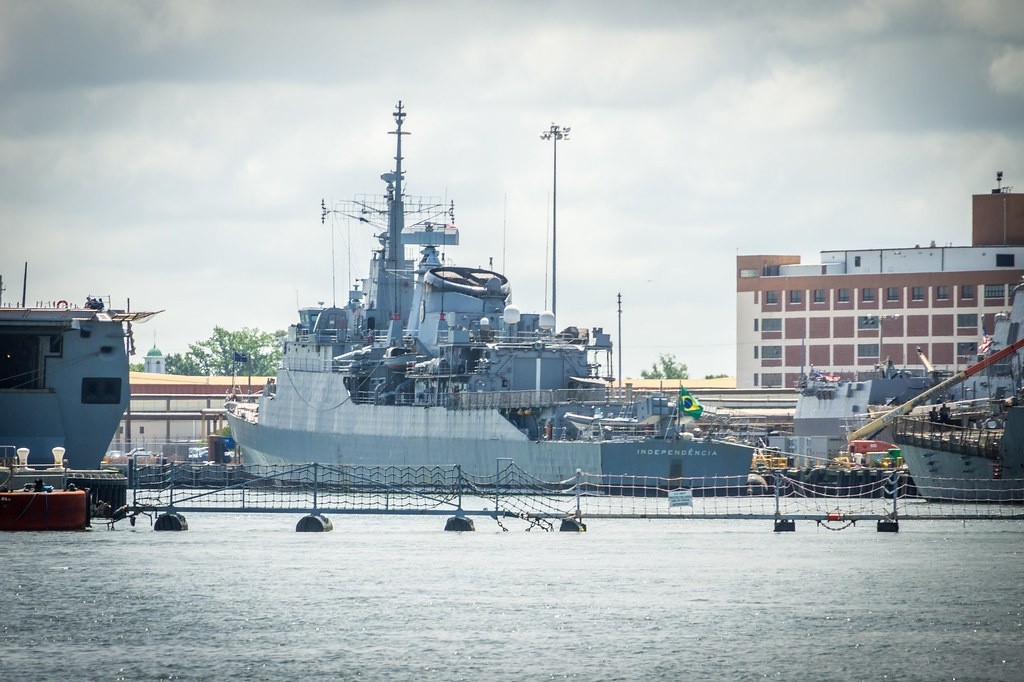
[980,326,991,353]
[233,349,247,362]
[679,386,703,420]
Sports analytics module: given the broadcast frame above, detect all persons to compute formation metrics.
[940,402,950,423]
[930,407,941,432]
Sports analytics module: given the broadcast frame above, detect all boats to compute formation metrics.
[224,100,754,497]
[892,400,1024,506]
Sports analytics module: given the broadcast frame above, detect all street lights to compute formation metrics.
[537,123,568,324]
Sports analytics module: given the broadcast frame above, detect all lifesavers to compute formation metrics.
[56,300,69,309]
